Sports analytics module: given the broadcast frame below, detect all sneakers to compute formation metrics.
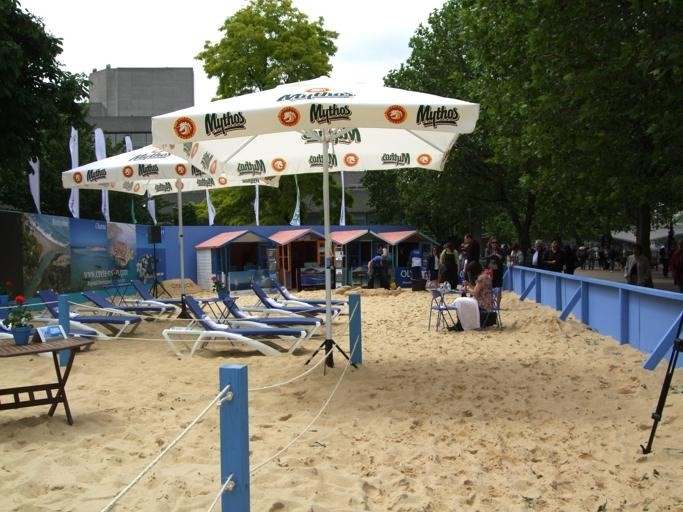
[450,322,464,331]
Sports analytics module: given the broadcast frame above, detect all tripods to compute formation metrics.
[640,309,683,454]
[149,244,172,298]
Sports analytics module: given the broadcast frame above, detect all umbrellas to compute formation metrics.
[149,76,484,338]
[60,142,282,300]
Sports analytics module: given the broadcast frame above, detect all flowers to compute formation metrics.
[1,292,32,330]
[210,276,223,293]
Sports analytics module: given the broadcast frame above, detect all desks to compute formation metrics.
[0,335,94,425]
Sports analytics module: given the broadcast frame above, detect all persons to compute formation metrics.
[625,244,641,284]
[410,234,480,296]
[368,246,392,290]
[651,239,682,289]
[444,258,495,332]
[485,236,506,309]
[501,237,575,274]
[566,242,633,272]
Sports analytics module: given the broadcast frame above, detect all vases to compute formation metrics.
[11,327,30,345]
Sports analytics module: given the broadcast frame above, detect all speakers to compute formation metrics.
[0,209,25,295]
[147,226,160,242]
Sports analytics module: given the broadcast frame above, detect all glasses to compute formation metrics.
[492,242,497,244]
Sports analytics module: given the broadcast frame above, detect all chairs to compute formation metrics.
[37,278,240,341]
[427,287,503,332]
[161,278,347,356]
